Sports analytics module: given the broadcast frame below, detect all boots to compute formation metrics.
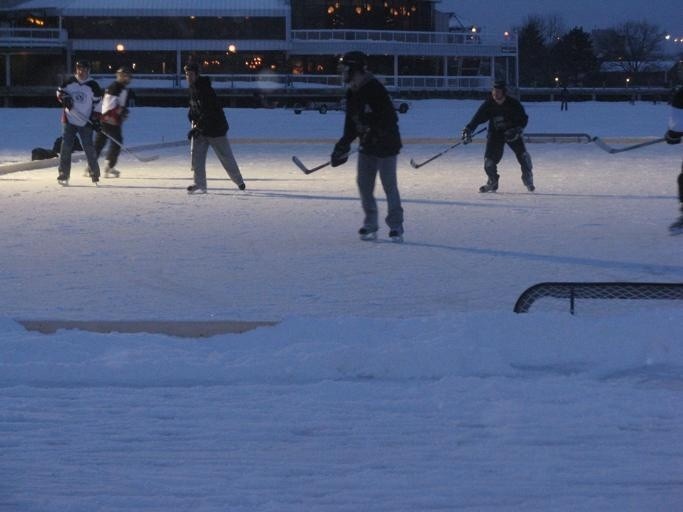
[480,175,499,191]
[521,173,535,191]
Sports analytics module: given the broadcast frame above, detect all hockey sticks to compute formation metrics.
[293,146,364,175]
[71,105,158,163]
[592,136,665,154]
[188,121,196,173]
[410,126,487,169]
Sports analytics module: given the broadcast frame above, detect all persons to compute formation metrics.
[663,61,682,234]
[127,87,135,107]
[560,86,568,110]
[461,79,535,193]
[331,50,404,238]
[56,57,100,183]
[181,62,244,191]
[87,65,132,174]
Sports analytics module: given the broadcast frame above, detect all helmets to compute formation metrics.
[117,66,130,83]
[339,50,369,82]
[76,60,90,79]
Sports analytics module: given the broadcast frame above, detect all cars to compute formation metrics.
[335,94,414,115]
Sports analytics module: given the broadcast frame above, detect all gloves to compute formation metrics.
[92,119,101,132]
[461,126,474,144]
[332,143,350,166]
[188,127,200,139]
[504,128,520,142]
[60,95,73,110]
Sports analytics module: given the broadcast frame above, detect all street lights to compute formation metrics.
[227,44,236,90]
[661,30,670,85]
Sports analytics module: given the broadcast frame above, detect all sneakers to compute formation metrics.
[104,166,120,174]
[359,222,404,237]
[187,184,206,191]
